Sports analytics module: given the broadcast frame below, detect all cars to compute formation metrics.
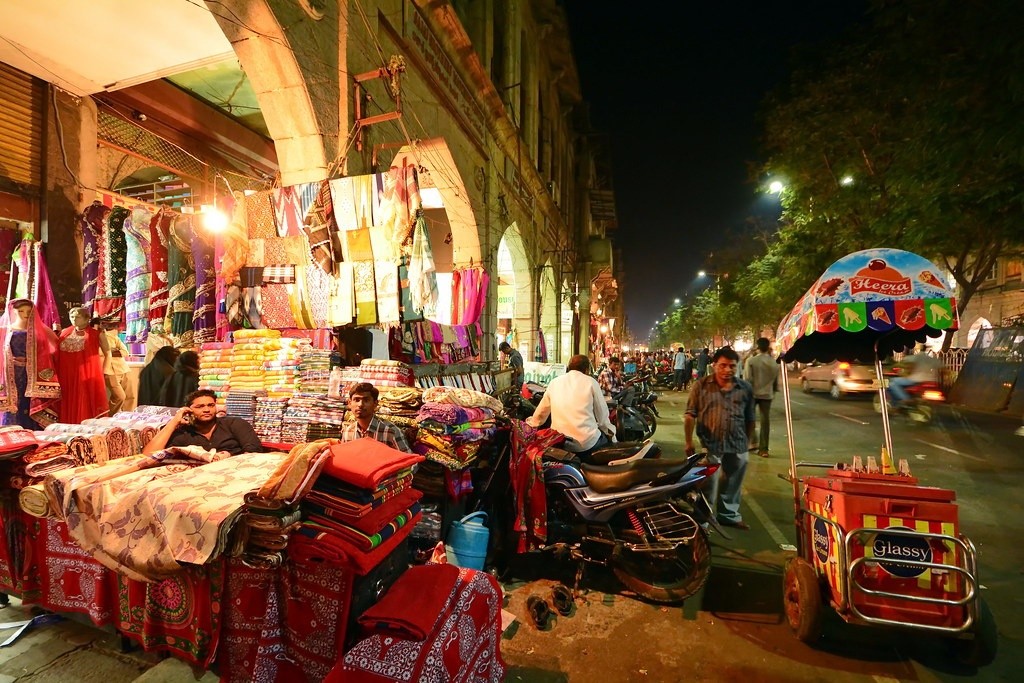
[800,357,880,400]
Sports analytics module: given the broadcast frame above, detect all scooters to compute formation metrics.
[520,349,689,443]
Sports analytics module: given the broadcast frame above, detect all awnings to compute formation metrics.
[772,247,962,557]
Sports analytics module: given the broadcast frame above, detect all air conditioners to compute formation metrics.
[546,180,561,210]
[592,240,613,278]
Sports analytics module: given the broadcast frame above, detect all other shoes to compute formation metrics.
[758,451,769,457]
[717,516,750,530]
[747,441,759,450]
[625,406,635,413]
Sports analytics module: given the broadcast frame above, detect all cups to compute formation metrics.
[898,458,912,478]
[867,456,879,475]
[853,456,865,474]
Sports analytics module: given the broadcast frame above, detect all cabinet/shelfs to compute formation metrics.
[112,179,203,214]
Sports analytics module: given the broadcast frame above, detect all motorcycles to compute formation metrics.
[471,401,731,604]
[868,357,945,428]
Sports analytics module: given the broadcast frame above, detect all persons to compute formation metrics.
[889,346,943,399]
[137,345,200,408]
[498,342,524,390]
[341,383,419,475]
[0,299,135,430]
[744,338,779,457]
[598,346,780,399]
[526,355,618,456]
[141,389,262,456]
[684,348,760,538]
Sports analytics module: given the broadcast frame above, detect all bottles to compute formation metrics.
[444,510,491,570]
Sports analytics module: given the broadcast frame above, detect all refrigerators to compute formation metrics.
[802,476,963,629]
